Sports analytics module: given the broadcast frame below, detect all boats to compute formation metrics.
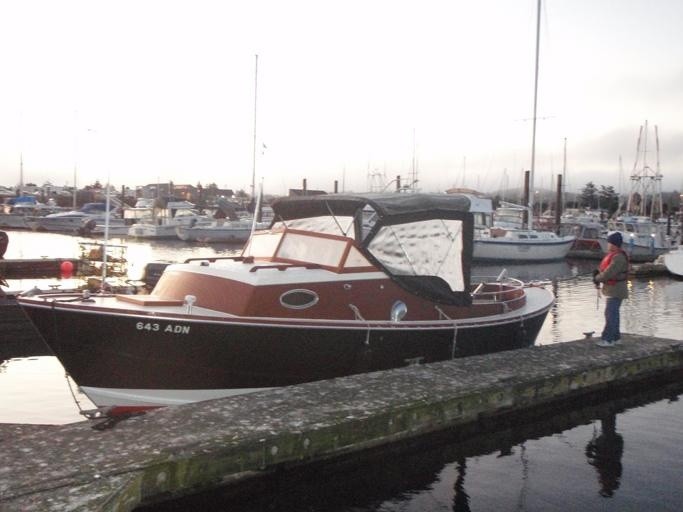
[470,0,683,279]
[18,193,558,419]
[0,53,274,242]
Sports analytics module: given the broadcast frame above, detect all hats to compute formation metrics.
[607,232,623,248]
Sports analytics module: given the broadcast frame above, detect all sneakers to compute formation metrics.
[597,339,622,348]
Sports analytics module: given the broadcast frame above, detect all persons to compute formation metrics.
[586,412,623,499]
[593,232,634,348]
